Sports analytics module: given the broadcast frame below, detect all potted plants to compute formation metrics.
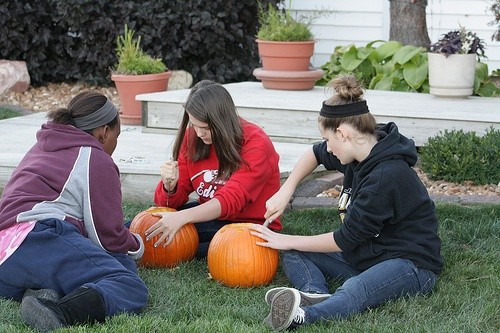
[110,23,172,125]
[426,22,488,97]
[251,0,324,91]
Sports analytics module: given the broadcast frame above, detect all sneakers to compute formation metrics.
[265,286,332,309]
[262,288,305,333]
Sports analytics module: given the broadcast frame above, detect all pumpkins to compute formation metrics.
[129,206,199,268]
[208,223,279,287]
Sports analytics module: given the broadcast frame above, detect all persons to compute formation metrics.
[258,75,443,332]
[118,79,283,260]
[0,88,148,333]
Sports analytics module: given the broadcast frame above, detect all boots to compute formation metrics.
[21,288,105,333]
[22,288,60,302]
